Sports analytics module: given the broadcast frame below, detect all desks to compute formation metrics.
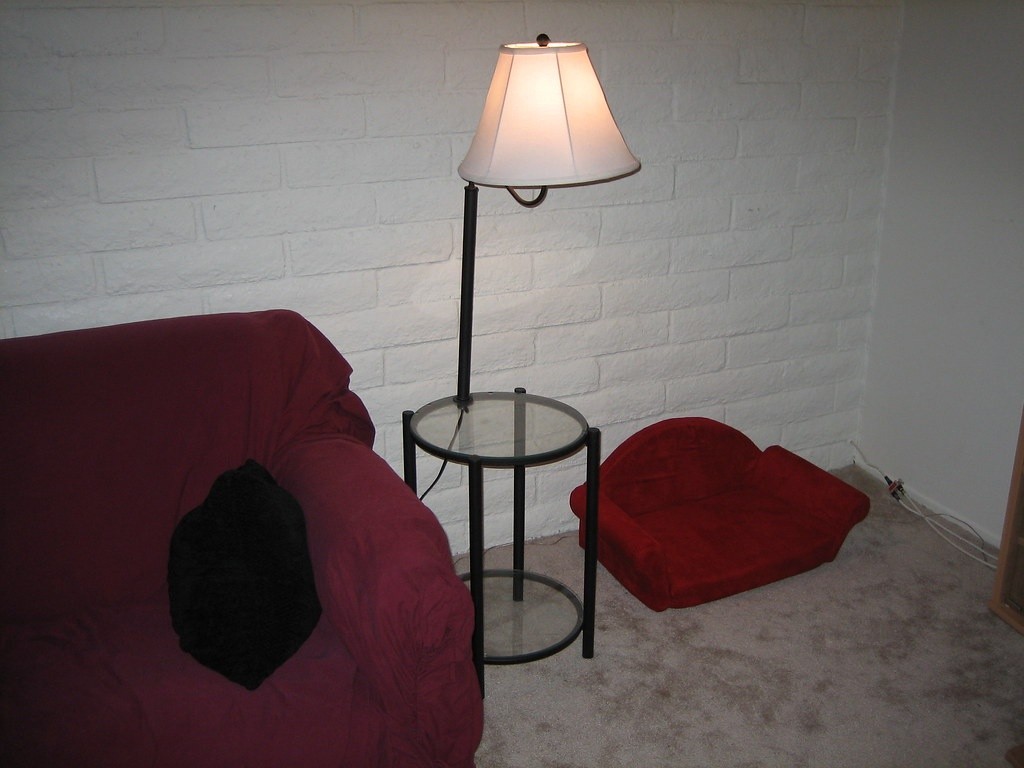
[401,386,601,696]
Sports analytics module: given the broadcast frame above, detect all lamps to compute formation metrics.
[453,33,641,410]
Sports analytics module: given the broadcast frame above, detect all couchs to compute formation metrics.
[0,308,484,768]
[570,417,871,611]
